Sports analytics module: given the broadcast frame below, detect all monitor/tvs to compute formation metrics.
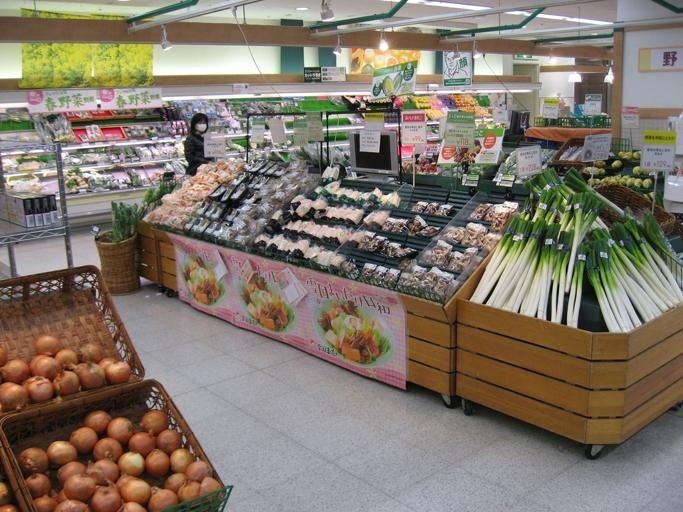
[349,131,398,180]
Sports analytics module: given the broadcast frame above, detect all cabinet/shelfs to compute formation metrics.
[174,97,354,159]
[454,297,683,446]
[136,218,503,398]
[1,113,190,196]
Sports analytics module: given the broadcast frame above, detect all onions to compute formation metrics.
[0,336,131,412]
[18,410,222,511]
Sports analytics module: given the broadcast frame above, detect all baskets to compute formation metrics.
[96,229,139,295]
[0,379,226,512]
[0,265,145,417]
[591,183,676,236]
[552,137,593,163]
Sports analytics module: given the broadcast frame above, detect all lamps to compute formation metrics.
[379,27,388,51]
[333,32,342,55]
[569,5,583,83]
[320,0,334,20]
[161,24,172,51]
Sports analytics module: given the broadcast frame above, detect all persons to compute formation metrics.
[184,112,216,179]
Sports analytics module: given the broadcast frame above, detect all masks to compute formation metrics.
[195,124,206,132]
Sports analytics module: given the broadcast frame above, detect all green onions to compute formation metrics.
[470,167,682,334]
